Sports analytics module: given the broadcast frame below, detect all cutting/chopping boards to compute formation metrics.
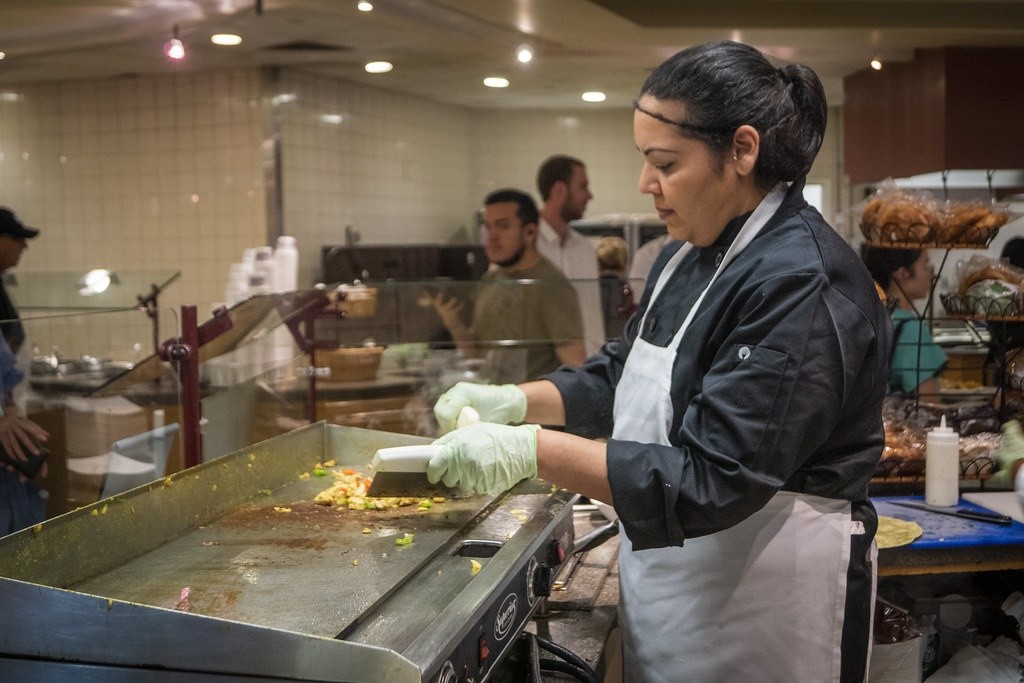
[870,495,1024,548]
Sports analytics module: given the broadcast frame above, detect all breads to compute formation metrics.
[878,419,995,477]
[958,262,1024,314]
[858,193,1010,245]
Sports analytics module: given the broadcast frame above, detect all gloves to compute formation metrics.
[426,406,540,496]
[434,381,528,433]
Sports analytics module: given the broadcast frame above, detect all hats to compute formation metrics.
[0,205,39,239]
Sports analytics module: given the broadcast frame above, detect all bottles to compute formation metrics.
[924,414,960,507]
[273,234,299,291]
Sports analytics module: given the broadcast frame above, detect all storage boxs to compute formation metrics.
[865,631,925,683]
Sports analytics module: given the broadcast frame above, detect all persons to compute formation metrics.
[427,39,886,683]
[595,236,634,340]
[0,331,49,536]
[534,154,605,361]
[434,188,586,387]
[0,207,40,356]
[859,243,951,402]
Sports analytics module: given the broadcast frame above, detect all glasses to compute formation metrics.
[911,263,934,276]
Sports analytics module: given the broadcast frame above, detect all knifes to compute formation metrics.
[888,501,1013,525]
